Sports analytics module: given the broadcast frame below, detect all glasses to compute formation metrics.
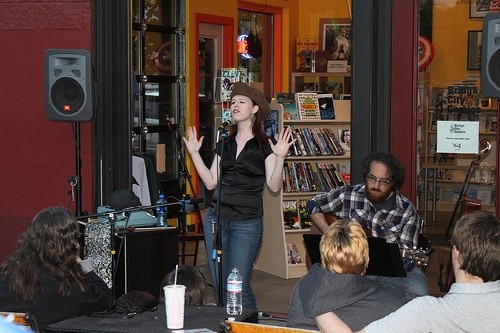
[366,175,392,186]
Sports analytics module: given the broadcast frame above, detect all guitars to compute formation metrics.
[305,213,437,272]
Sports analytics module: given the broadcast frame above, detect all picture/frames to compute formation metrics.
[467,30,483,70]
[318,17,352,60]
[471,0,500,17]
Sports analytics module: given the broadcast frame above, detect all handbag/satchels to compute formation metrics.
[114,290,158,318]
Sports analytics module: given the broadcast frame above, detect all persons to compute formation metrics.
[313,210,500,333]
[159,265,207,307]
[286,220,411,333]
[308,152,429,298]
[0,208,114,333]
[182,81,297,309]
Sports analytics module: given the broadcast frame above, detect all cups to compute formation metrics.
[163,285,186,330]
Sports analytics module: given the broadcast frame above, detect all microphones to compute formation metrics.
[184,197,205,204]
[218,116,235,130]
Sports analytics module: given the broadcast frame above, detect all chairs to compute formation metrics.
[166,196,209,267]
[1,311,42,333]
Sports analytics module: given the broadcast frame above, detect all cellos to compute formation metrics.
[436,137,493,293]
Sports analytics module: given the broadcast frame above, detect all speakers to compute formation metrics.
[45,48,94,122]
[480,12,500,98]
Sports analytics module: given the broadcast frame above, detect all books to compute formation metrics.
[276,92,336,121]
[279,124,350,156]
[282,161,351,191]
[284,198,321,229]
[215,67,247,100]
[417,72,497,202]
[295,37,320,72]
[286,240,301,264]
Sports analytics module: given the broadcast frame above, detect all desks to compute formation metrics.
[46,301,257,333]
[113,228,178,301]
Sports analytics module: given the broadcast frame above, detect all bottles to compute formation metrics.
[157,195,167,227]
[226,268,242,315]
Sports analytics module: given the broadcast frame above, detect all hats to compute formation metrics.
[231,81,270,121]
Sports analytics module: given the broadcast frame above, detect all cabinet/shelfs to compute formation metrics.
[258,98,351,278]
[420,87,496,213]
[290,71,352,100]
